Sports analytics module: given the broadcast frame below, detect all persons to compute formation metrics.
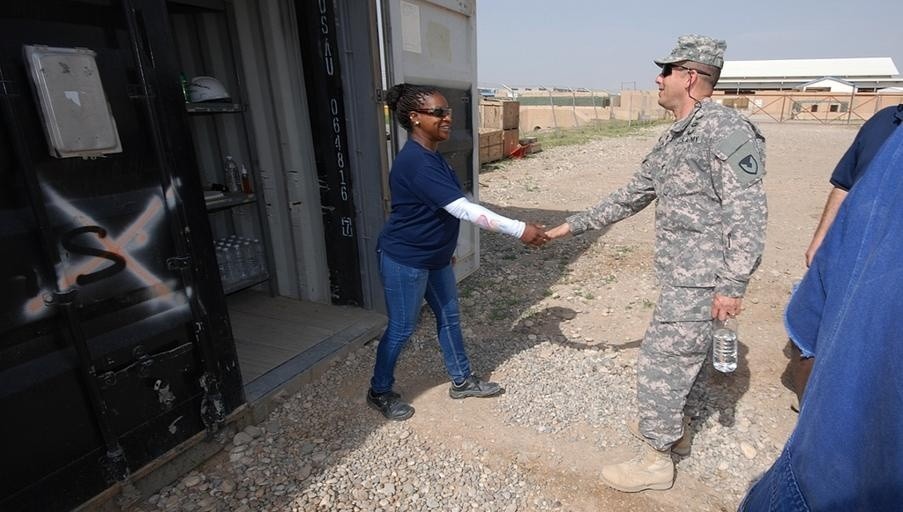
[363,83,551,420]
[805,104,902,268]
[550,31,769,492]
[735,129,902,511]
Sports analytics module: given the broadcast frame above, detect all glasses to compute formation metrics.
[407,106,452,119]
[661,63,711,77]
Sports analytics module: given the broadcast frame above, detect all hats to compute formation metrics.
[653,34,726,70]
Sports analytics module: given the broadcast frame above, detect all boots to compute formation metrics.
[627,413,693,455]
[600,441,674,493]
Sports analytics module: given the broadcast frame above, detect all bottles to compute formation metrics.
[710,313,739,374]
[210,233,267,285]
[223,154,244,196]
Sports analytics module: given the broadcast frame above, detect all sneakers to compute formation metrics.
[449,375,501,398]
[367,386,415,420]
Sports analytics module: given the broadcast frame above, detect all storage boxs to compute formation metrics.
[479,97,520,166]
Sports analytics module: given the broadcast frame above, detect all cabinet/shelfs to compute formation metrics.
[159,0,280,298]
